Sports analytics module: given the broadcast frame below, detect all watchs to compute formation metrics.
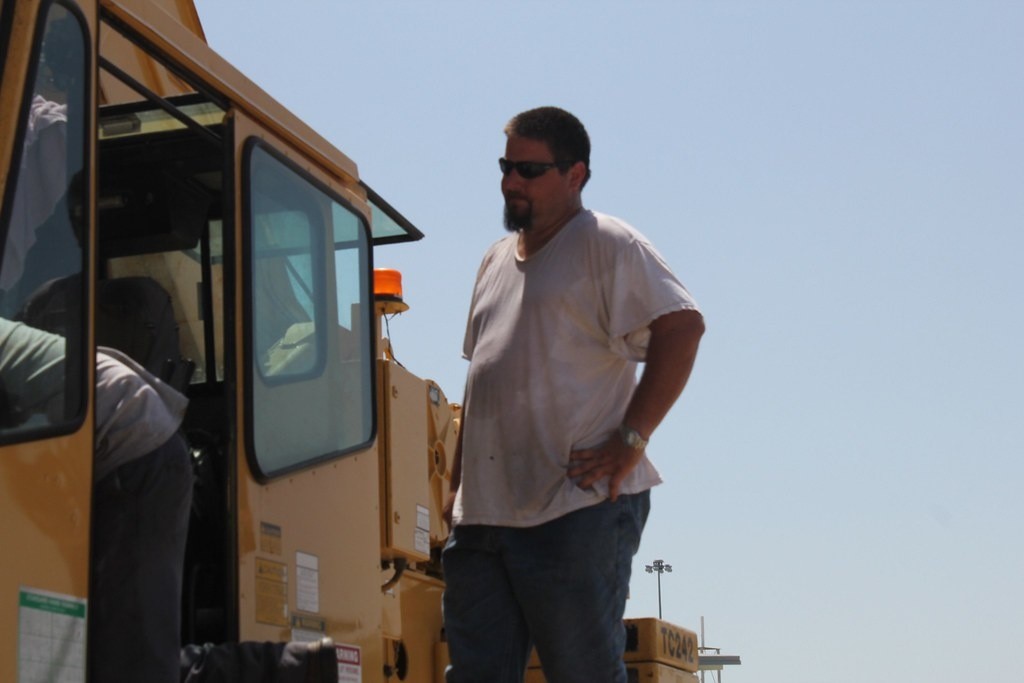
[616,422,651,453]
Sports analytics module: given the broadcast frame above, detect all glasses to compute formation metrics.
[499,158,562,180]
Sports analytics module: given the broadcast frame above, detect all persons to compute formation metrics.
[437,104,707,683]
[0,38,340,682]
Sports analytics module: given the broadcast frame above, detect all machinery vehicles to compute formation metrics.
[0,0,744,682]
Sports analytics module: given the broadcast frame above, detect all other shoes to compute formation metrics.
[306,637,339,683]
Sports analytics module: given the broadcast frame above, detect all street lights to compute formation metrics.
[644,557,673,621]
